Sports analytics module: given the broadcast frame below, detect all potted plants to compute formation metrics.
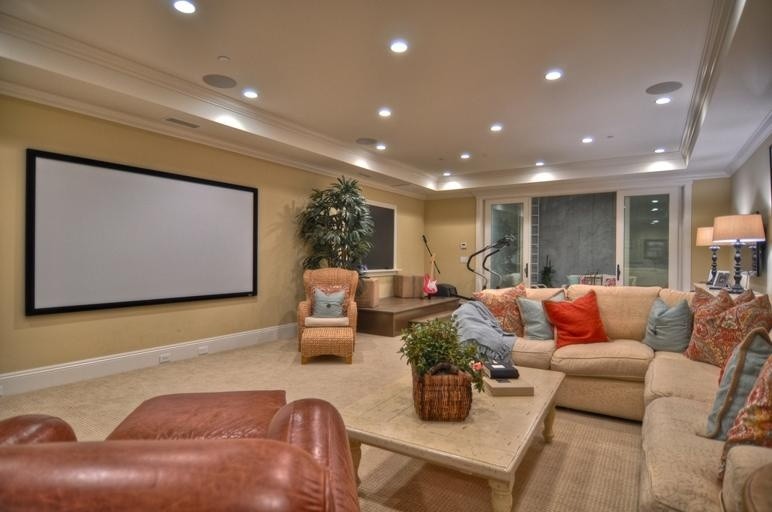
[395,313,490,422]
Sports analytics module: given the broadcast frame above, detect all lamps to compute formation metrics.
[712,214,767,293]
[695,226,728,285]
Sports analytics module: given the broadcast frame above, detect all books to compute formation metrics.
[481,374,535,398]
[482,361,520,379]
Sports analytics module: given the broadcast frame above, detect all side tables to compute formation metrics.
[744,463,772,512]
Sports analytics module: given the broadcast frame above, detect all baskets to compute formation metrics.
[411,359,472,421]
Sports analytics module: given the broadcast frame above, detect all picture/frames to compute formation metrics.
[712,271,731,287]
[644,239,668,260]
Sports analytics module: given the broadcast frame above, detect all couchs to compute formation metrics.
[453,287,772,512]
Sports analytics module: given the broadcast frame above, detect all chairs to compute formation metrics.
[296,267,359,365]
[578,271,603,285]
[0,389,359,512]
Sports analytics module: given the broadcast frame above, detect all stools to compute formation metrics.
[393,274,428,298]
[438,284,457,297]
[355,276,379,308]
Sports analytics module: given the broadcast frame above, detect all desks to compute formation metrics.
[565,274,639,286]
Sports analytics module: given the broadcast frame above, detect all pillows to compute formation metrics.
[310,285,350,318]
